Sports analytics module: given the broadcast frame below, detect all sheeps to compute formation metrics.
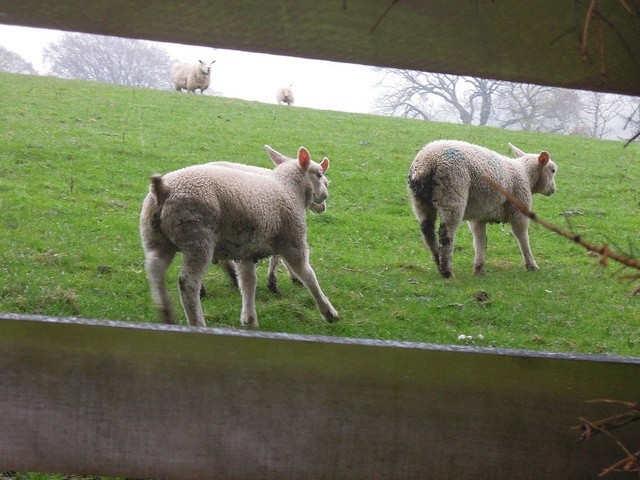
[171,57,217,94]
[276,83,294,106]
[406,139,558,280]
[138,146,341,329]
[199,144,330,299]
